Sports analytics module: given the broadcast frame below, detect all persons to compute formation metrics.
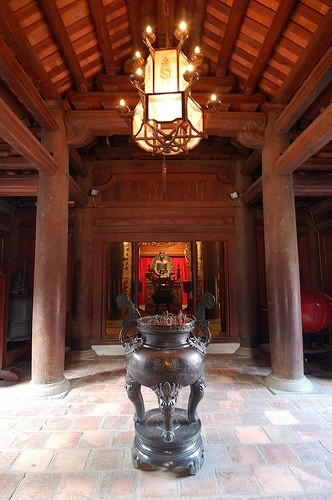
[154,252,170,278]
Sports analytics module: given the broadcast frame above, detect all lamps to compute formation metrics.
[114,0,223,158]
